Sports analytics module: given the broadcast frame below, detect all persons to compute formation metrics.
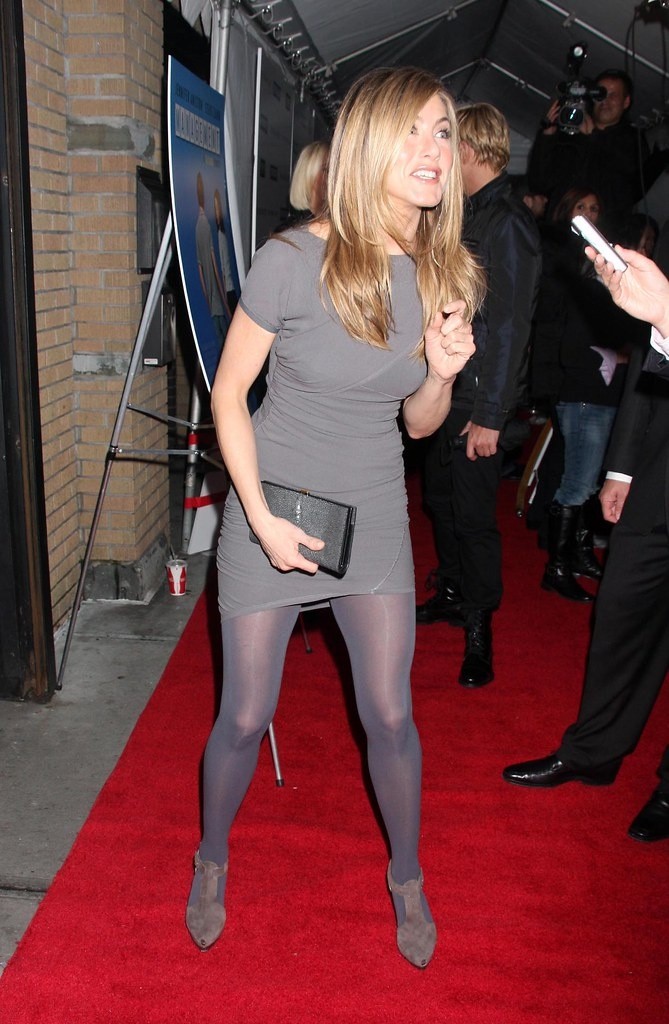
[186,67,479,970]
[288,69,669,842]
[195,171,238,359]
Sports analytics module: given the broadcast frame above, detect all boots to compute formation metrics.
[526,481,571,551]
[541,498,596,604]
[566,494,604,583]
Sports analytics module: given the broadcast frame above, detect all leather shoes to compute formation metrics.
[458,608,493,688]
[416,570,469,627]
[501,750,614,788]
[628,780,669,842]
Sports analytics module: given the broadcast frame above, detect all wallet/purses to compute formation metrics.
[249,481,358,574]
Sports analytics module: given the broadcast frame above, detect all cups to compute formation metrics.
[166,560,187,596]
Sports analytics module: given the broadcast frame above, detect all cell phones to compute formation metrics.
[570,213,629,273]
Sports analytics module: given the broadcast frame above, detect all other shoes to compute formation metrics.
[387,858,437,968]
[502,464,540,480]
[185,849,228,949]
